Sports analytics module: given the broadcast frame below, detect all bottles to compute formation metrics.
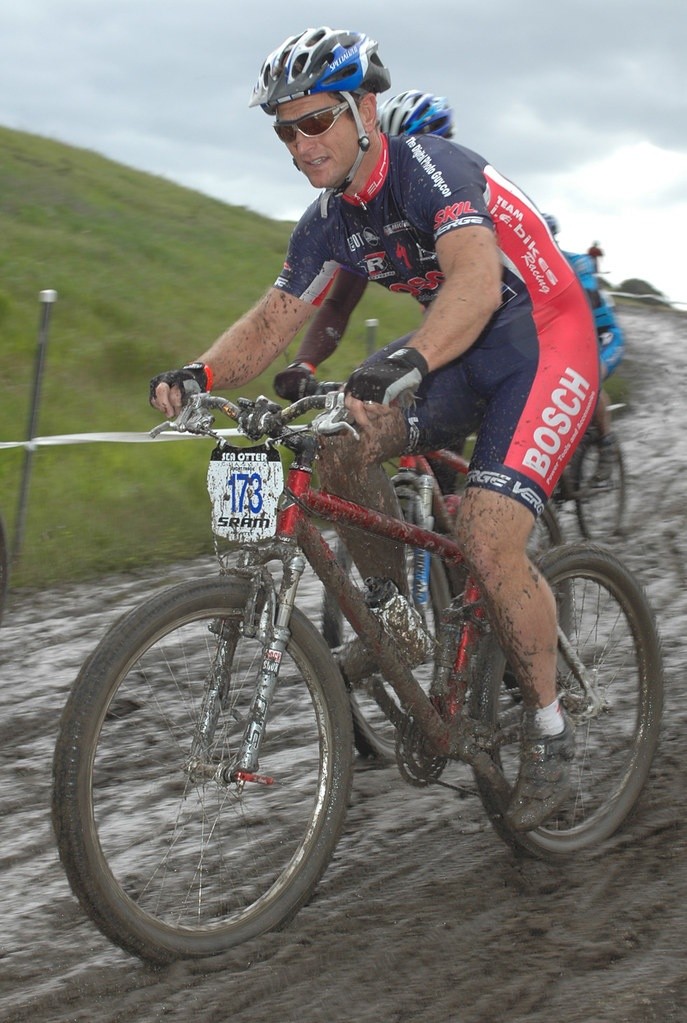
[363,577,435,669]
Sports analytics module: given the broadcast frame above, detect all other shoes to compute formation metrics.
[587,446,617,488]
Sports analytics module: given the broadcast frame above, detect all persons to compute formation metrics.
[149,25,625,830]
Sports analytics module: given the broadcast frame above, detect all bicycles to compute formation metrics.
[293,375,629,762]
[53,378,662,961]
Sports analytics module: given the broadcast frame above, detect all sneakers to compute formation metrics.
[505,708,577,831]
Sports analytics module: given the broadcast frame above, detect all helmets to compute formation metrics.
[249,26,390,115]
[378,90,453,136]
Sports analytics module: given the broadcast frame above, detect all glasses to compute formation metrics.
[273,100,349,142]
